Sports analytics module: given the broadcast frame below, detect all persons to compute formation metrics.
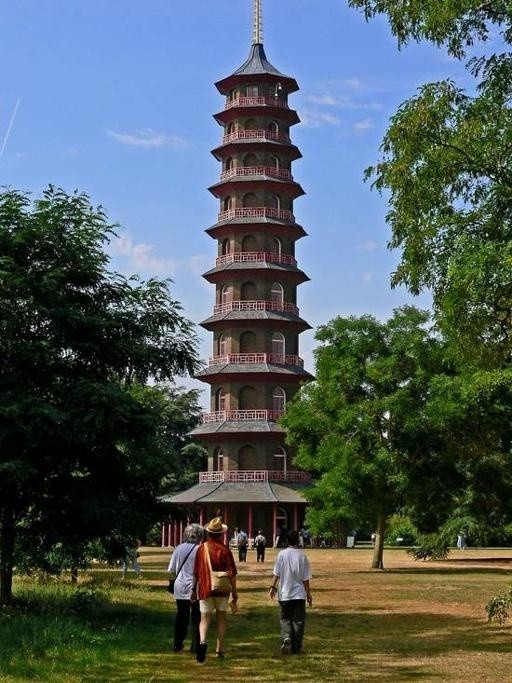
[121,539,143,581]
[268,530,312,654]
[166,523,204,653]
[457,534,465,550]
[371,530,378,545]
[233,526,327,563]
[190,519,238,664]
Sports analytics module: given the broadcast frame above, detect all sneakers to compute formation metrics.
[281,637,291,654]
[174,644,183,651]
[121,574,145,580]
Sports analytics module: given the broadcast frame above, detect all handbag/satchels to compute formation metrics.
[210,571,232,593]
[167,579,174,594]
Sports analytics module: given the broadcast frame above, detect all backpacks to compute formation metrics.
[257,536,265,550]
[239,533,248,546]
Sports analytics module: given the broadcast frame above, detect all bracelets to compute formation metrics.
[268,586,278,593]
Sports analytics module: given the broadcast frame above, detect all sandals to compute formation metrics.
[216,651,225,658]
[196,641,208,662]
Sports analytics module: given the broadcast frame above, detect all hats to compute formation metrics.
[204,517,228,534]
